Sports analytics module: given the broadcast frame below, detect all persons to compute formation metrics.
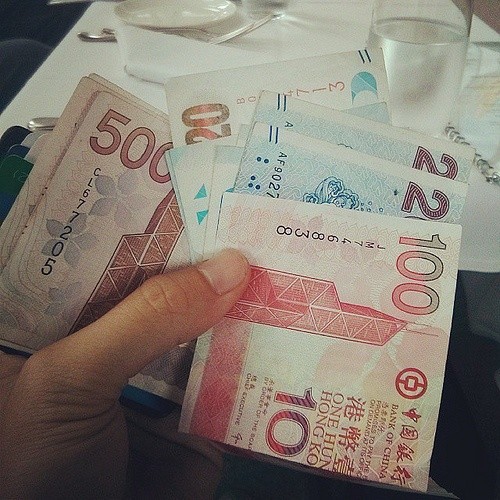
[1,247,252,500]
[0,37,54,113]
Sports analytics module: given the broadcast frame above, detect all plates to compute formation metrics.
[114,0,237,28]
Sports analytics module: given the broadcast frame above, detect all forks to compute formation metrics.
[99,14,275,41]
[76,25,253,44]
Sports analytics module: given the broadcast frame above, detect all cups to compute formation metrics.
[367,0,476,137]
[242,0,290,20]
